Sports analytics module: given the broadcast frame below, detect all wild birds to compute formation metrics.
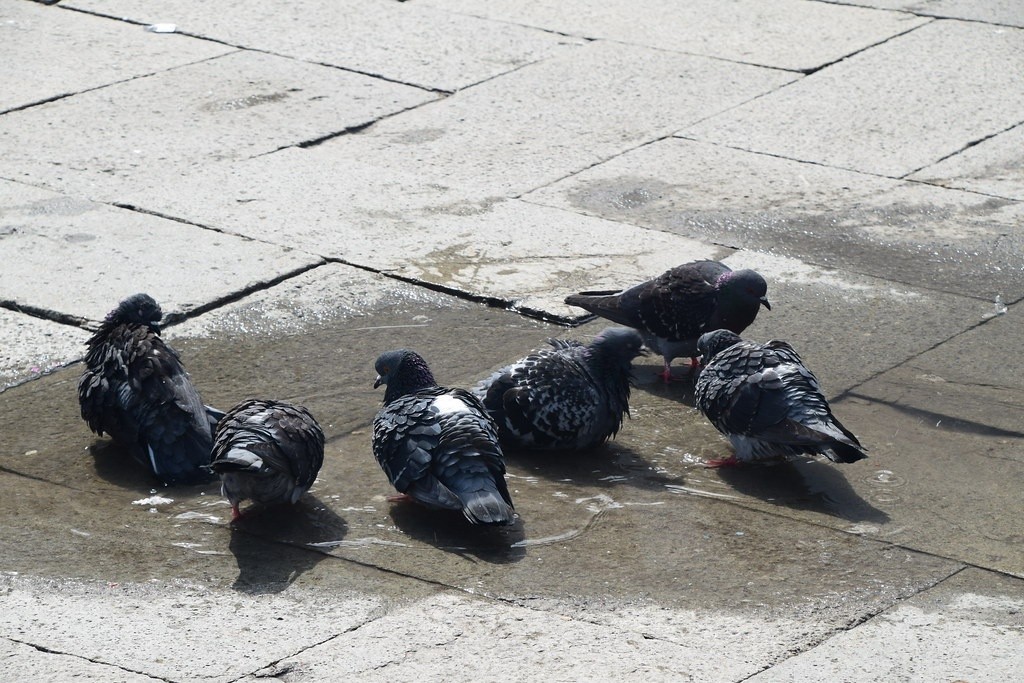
[210,396,325,522]
[563,257,773,390]
[370,349,519,526]
[474,326,654,461]
[77,292,228,481]
[695,329,871,475]
